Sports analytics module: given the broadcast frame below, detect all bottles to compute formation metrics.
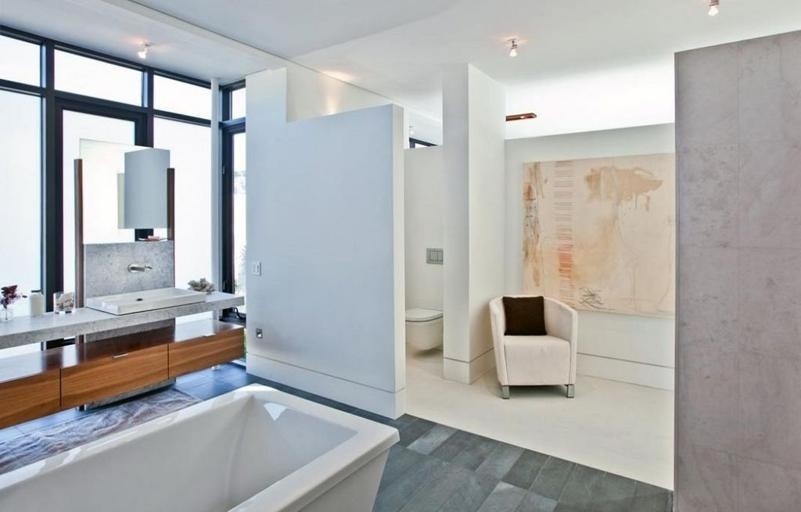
[28,290,45,317]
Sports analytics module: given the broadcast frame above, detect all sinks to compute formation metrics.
[88,287,208,314]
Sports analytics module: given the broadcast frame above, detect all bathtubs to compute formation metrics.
[0,383,400,510]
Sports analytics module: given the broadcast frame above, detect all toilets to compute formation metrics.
[405,309,442,352]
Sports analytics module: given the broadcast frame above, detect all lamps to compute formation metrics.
[117,149,169,241]
[708,0,720,15]
[138,43,154,58]
[509,39,518,56]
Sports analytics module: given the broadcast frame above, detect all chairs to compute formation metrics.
[489,294,579,399]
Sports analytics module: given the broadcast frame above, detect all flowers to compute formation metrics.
[0,285,20,320]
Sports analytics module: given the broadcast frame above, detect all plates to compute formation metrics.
[187,287,216,296]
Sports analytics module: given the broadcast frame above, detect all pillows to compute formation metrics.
[503,296,548,336]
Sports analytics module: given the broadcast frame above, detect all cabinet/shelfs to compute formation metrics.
[0,289,245,428]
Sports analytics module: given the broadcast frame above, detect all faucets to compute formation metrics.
[129,264,153,273]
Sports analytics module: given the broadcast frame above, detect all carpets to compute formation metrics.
[0,388,203,474]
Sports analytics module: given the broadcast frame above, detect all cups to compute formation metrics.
[52,291,74,315]
